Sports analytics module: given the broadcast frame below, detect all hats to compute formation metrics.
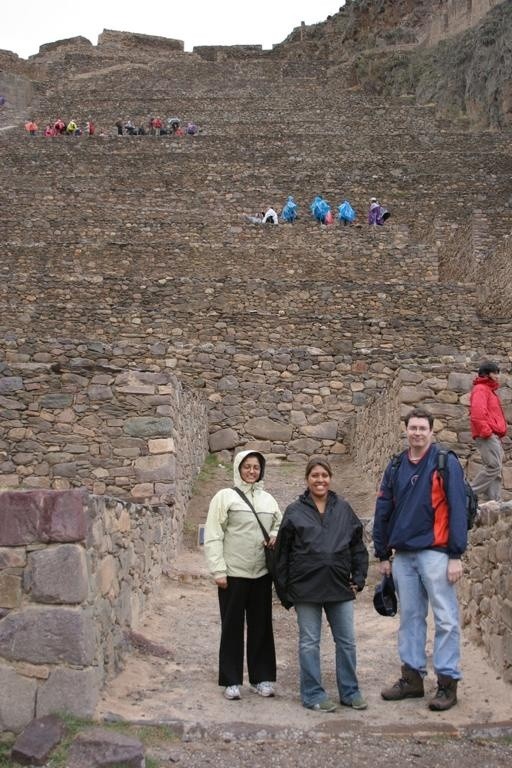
[374,571,397,616]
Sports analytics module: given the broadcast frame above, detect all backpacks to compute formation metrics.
[387,449,477,530]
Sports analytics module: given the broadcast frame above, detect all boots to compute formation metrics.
[381,665,424,700]
[429,673,457,711]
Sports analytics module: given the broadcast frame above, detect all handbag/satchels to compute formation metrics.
[264,546,275,569]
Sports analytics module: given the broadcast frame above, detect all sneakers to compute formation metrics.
[352,698,368,709]
[311,700,337,712]
[224,685,241,700]
[248,681,275,697]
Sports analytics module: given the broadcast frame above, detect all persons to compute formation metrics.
[373,408,469,710]
[204,448,283,702]
[261,190,390,225]
[26,112,198,137]
[272,457,369,712]
[468,359,508,502]
